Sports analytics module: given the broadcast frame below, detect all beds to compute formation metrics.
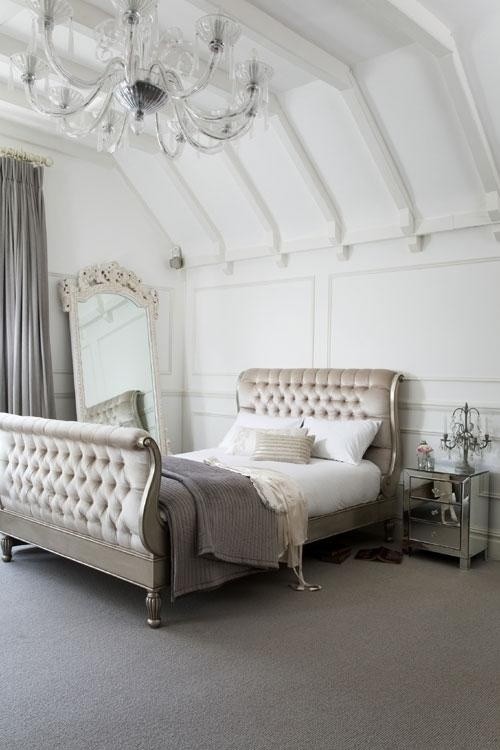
[0,367,407,630]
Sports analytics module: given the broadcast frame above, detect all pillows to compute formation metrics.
[214,410,384,465]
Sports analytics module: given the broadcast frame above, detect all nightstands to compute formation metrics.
[402,463,490,571]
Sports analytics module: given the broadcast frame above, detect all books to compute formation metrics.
[354,546,403,566]
[312,544,355,565]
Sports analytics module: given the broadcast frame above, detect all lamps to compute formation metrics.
[439,402,495,472]
[9,1,276,161]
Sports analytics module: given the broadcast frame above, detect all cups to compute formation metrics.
[426,447,435,469]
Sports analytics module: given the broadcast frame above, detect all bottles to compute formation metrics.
[417,440,429,468]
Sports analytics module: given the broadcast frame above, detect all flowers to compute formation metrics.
[416,440,434,466]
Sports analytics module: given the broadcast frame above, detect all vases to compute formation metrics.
[418,454,430,471]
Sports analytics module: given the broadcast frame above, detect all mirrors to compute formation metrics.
[56,261,167,458]
